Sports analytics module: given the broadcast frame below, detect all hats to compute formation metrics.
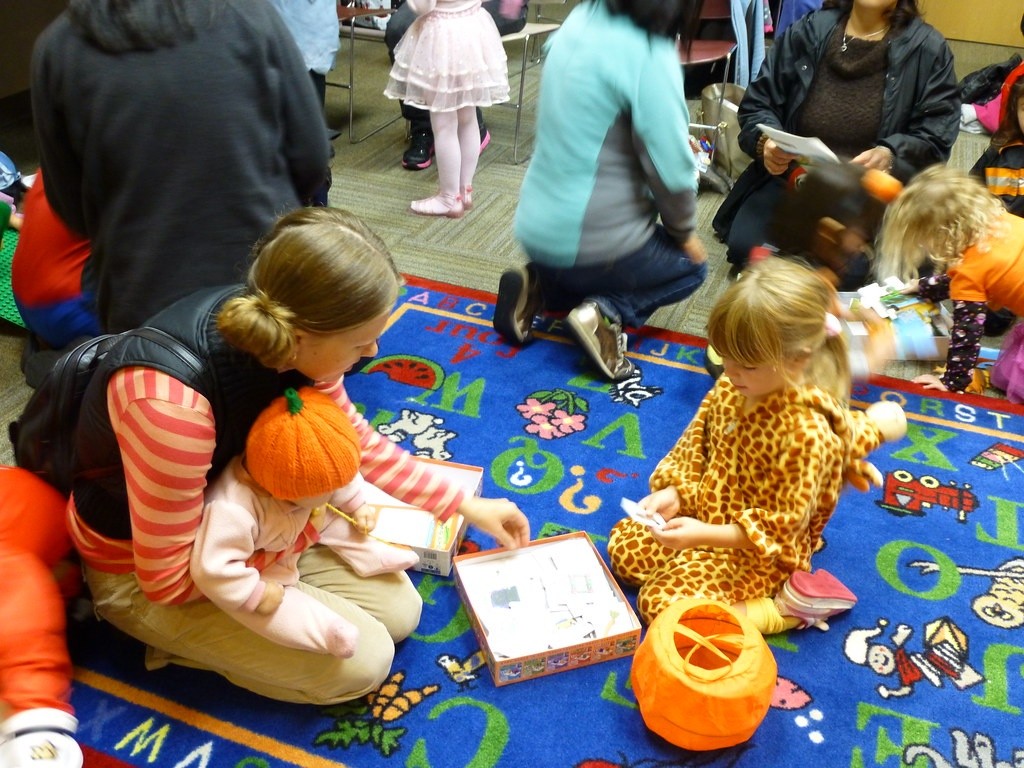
[246,386,362,501]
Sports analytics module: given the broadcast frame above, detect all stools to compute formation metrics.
[323,6,403,144]
[492,22,559,164]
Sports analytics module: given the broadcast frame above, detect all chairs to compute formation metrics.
[674,0,766,152]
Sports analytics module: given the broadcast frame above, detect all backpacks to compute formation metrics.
[9,326,224,496]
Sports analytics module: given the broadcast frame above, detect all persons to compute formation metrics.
[0,0,344,387]
[880,167,1024,405]
[66,207,531,706]
[190,387,419,658]
[607,259,907,633]
[382,0,511,216]
[493,0,710,379]
[713,0,960,286]
[915,75,1024,336]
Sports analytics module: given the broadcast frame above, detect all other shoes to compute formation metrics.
[410,194,464,218]
[25,336,93,391]
[461,187,473,209]
[326,127,342,141]
[20,338,48,373]
[329,145,336,157]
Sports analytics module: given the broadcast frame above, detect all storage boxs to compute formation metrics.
[332,457,490,577]
[454,529,642,683]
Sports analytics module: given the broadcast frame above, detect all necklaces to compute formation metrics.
[840,25,887,52]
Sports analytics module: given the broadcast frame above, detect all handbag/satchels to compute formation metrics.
[701,82,756,182]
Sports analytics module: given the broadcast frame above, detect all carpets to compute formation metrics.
[64,274,1024,768]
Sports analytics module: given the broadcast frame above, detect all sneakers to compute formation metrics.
[773,568,858,631]
[477,108,490,157]
[566,301,635,382]
[492,267,538,348]
[402,120,434,171]
